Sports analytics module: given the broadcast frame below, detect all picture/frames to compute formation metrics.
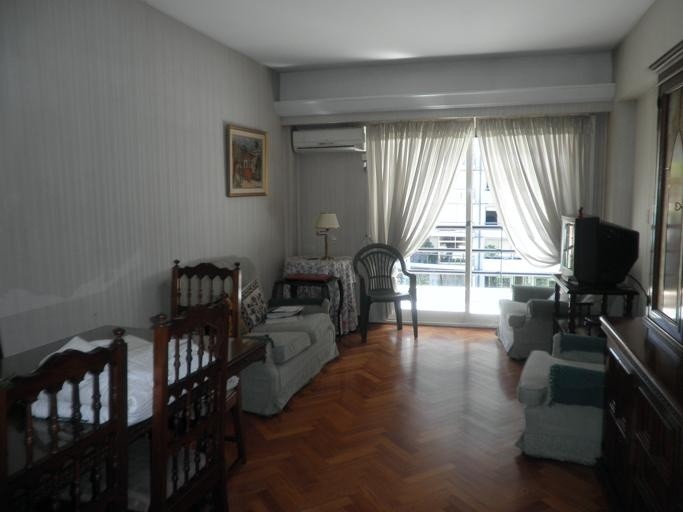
[227,124,268,196]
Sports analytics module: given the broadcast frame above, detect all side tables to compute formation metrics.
[270,256,356,336]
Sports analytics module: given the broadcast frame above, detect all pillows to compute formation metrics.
[241,277,268,330]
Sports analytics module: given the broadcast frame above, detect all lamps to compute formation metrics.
[314,213,341,260]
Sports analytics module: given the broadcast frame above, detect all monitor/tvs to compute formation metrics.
[560,214,640,290]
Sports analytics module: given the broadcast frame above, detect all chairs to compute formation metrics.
[353,243,419,343]
[497,285,625,466]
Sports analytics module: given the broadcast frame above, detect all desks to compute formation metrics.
[551,272,639,334]
[0,325,271,512]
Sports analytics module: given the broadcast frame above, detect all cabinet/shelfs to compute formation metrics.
[599,40,682,511]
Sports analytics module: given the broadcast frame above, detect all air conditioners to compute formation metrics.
[293,126,367,153]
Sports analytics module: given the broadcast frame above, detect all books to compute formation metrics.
[265,303,306,319]
[265,311,303,324]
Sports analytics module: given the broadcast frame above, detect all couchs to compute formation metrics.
[160,256,340,417]
